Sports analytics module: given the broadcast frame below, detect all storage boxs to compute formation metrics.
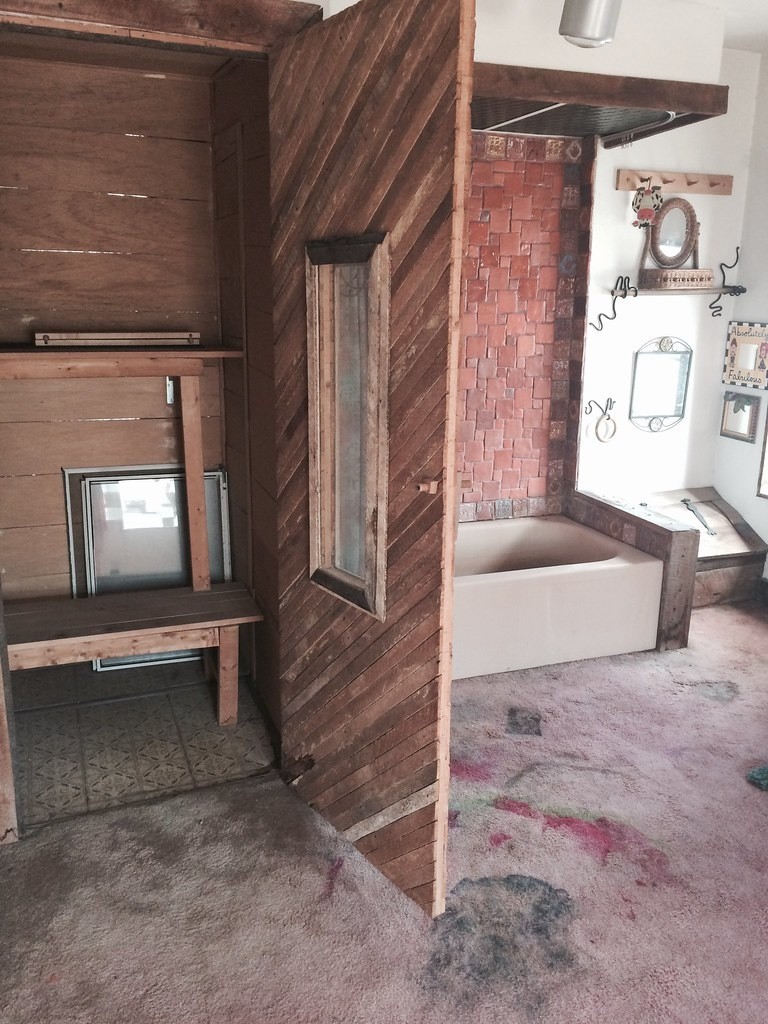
[630,486,768,612]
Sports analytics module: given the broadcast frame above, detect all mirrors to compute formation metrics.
[629,336,693,434]
[722,321,768,391]
[720,392,760,443]
[650,198,698,268]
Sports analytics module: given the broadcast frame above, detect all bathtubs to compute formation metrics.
[451,514,664,679]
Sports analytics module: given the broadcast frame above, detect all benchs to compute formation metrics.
[5,583,267,727]
